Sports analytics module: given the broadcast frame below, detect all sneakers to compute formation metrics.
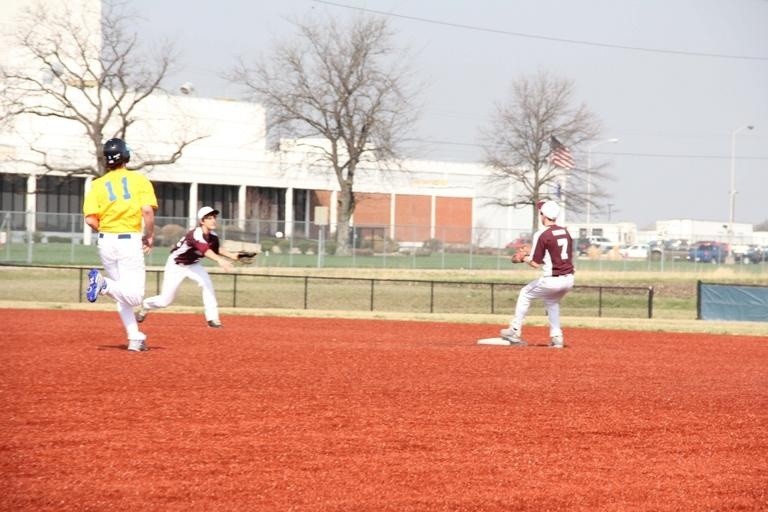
[135,306,146,322]
[87,270,103,302]
[128,340,147,352]
[551,336,564,348]
[500,328,522,343]
[208,320,222,327]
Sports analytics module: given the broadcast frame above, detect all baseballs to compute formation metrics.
[275,232,284,238]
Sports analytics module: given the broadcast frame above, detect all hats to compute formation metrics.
[536,199,560,220]
[198,207,220,219]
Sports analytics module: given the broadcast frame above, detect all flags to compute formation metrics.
[548,136,576,170]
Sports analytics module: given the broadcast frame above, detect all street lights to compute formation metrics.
[585,136,619,225]
[729,123,755,226]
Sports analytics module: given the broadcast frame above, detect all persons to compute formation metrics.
[135,205,256,328]
[83,138,159,352]
[500,200,575,348]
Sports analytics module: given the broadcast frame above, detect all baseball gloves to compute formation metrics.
[237,249,256,264]
[512,253,529,263]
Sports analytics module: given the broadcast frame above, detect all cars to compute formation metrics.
[505,236,532,252]
[572,235,767,264]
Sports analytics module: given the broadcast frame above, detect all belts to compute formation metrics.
[99,233,133,240]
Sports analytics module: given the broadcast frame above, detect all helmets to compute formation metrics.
[103,138,129,167]
